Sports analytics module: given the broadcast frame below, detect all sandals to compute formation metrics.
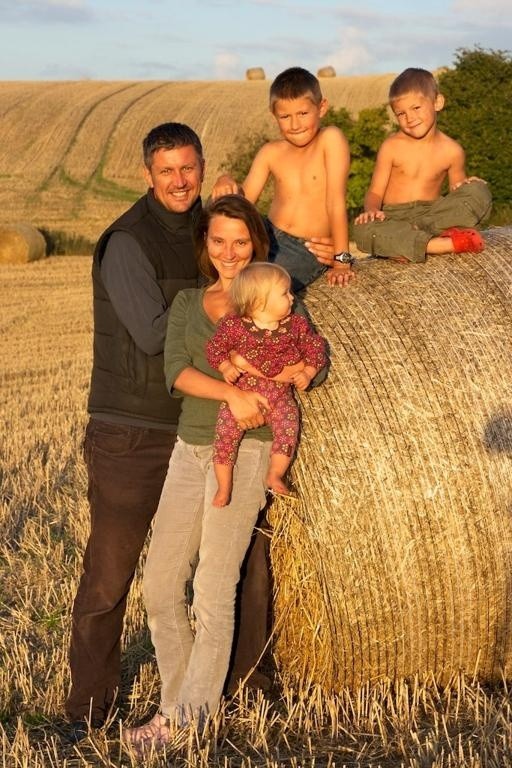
[438,227,486,253]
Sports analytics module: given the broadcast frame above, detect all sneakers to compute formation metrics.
[128,729,202,757]
[124,714,167,743]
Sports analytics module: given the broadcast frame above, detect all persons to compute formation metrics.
[354,67,495,263]
[64,123,334,744]
[123,194,330,760]
[200,262,330,508]
[199,65,357,289]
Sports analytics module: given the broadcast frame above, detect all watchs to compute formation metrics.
[334,252,355,266]
